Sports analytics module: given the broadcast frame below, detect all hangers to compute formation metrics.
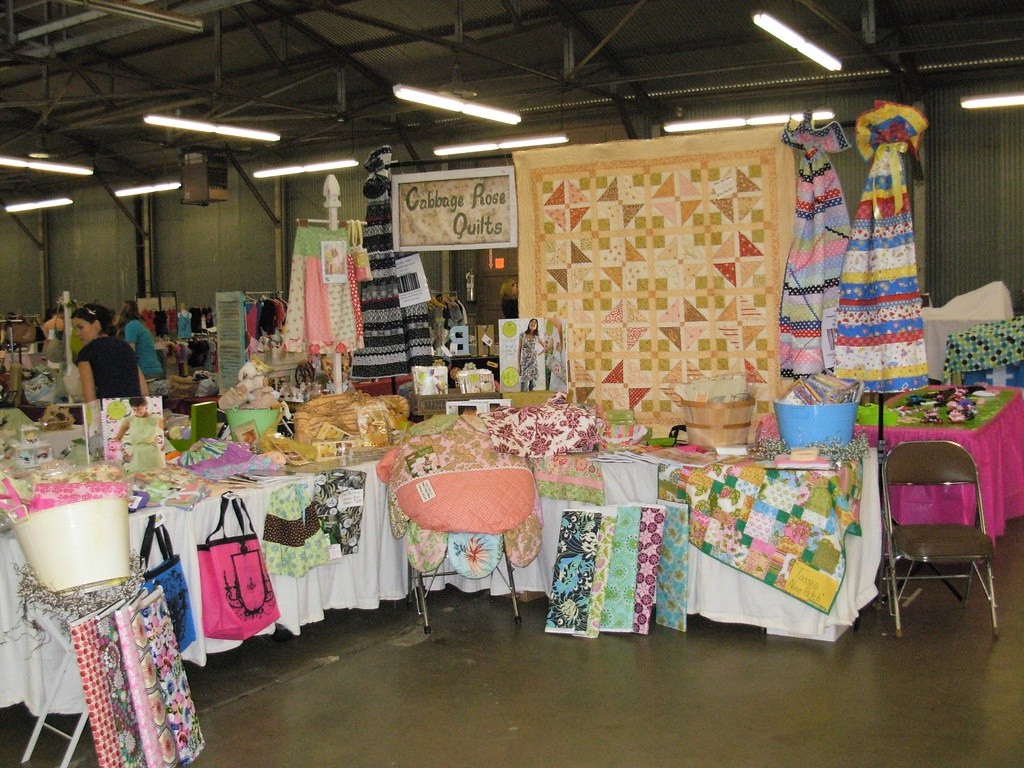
[242,290,288,311]
[139,305,218,351]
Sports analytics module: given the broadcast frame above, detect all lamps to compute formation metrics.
[0,0,1024,221]
[0,0,1024,215]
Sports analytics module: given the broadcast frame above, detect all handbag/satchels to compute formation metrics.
[7,316,48,345]
[196,491,280,641]
[139,514,197,653]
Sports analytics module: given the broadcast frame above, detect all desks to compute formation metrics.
[855,385,1024,566]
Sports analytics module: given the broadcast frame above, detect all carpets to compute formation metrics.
[423,288,466,317]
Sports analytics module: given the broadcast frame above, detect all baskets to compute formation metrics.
[39,417,75,431]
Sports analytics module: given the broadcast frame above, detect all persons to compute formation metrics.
[500,278,519,319]
[115,397,164,470]
[116,300,163,383]
[517,318,546,392]
[142,305,192,338]
[70,304,149,403]
[43,301,64,340]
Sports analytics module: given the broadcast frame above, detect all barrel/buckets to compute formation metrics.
[223,406,281,441]
[772,397,858,451]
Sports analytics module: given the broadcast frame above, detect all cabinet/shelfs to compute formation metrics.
[18,596,110,768]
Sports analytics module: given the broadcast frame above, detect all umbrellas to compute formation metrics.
[833,100,929,510]
[348,144,434,396]
[778,108,852,379]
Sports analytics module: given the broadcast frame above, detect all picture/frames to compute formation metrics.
[391,166,518,253]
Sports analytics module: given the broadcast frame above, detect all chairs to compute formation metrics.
[376,414,541,636]
[880,439,1001,639]
